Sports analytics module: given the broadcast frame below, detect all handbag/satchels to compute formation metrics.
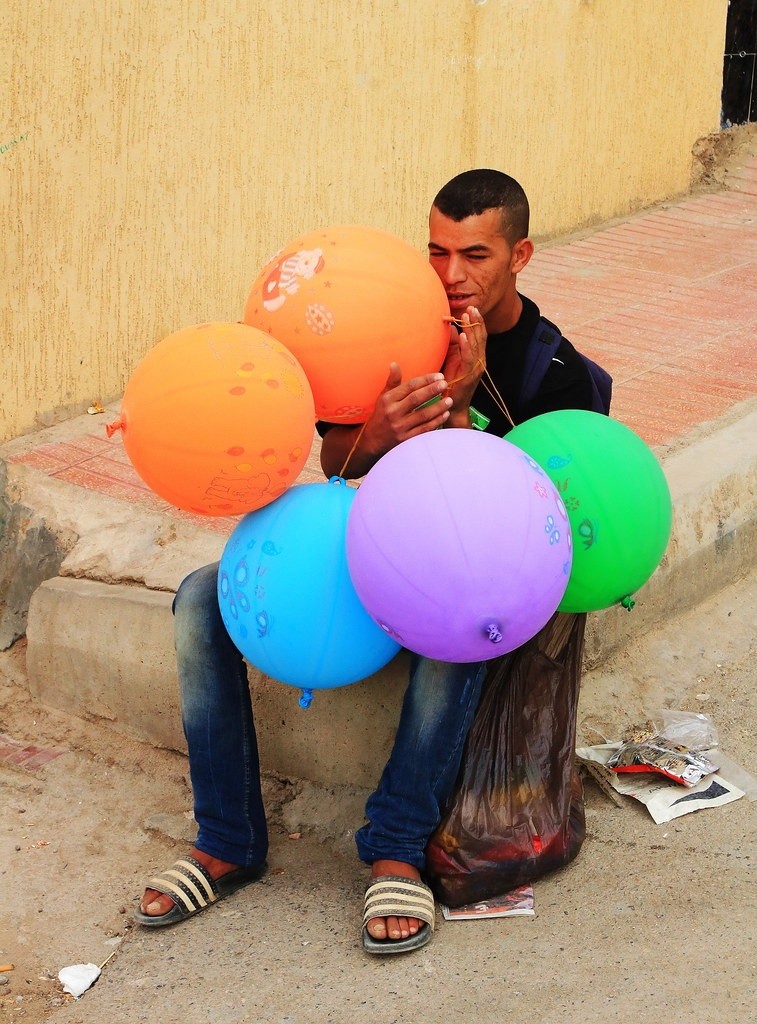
[423,610,587,909]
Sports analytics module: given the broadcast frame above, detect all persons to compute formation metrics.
[127,168,612,957]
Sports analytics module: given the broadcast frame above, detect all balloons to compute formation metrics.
[236,223,455,426]
[217,475,404,709]
[104,320,317,519]
[345,424,574,664]
[499,408,674,614]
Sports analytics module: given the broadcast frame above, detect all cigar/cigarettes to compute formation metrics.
[0,964,15,972]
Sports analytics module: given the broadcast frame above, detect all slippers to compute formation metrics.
[134,855,268,927]
[358,876,433,955]
